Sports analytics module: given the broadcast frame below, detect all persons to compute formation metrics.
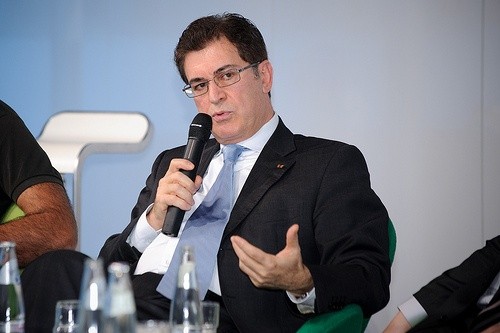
[383,235,500,333]
[19,12,391,333]
[0,99,77,269]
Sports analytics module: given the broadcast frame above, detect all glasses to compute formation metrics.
[182,61,263,98]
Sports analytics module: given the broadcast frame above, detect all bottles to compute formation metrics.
[98,262,137,332]
[74,258,107,333]
[169,245,202,333]
[0,240,25,333]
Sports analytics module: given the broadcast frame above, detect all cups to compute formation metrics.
[199,302,218,333]
[53,300,79,333]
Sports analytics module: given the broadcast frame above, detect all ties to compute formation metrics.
[156,143,248,302]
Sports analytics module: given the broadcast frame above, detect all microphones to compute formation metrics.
[162,113,213,238]
